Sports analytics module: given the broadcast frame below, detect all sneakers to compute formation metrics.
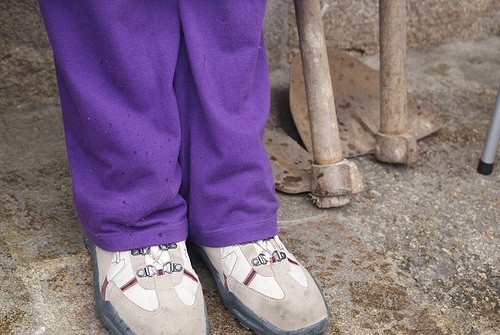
[87,238,209,335]
[187,234,329,335]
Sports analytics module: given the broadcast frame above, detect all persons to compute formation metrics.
[41,0,334,335]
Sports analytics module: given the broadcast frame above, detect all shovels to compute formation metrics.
[288,0,442,165]
